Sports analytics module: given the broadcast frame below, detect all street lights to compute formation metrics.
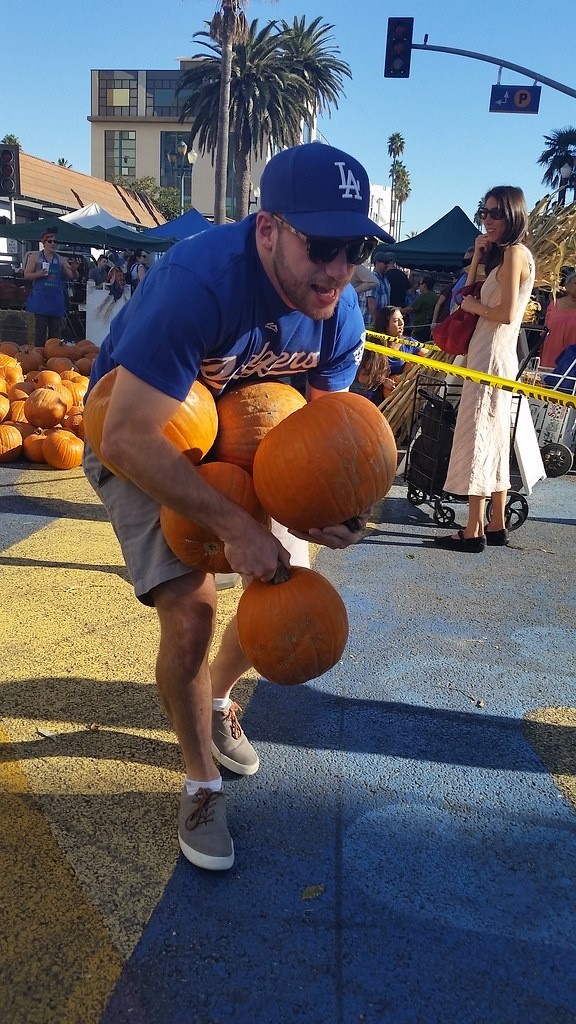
[167,140,198,216]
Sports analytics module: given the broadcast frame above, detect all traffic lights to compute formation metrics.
[0,144,21,197]
[384,16,414,78]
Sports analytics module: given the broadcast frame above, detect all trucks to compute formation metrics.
[0,250,97,296]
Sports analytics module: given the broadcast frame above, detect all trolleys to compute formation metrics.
[404,323,549,530]
[518,357,576,479]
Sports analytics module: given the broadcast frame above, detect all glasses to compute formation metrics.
[477,206,505,221]
[139,255,146,259]
[45,239,57,244]
[264,211,378,266]
[98,255,108,261]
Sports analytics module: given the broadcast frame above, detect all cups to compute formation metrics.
[42,262,50,275]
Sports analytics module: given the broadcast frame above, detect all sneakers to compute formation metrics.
[208,697,260,776]
[178,782,235,871]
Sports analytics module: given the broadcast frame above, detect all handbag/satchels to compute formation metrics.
[430,280,485,356]
[409,386,455,496]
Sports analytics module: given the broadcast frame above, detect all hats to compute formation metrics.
[559,266,576,286]
[418,275,437,288]
[371,252,390,264]
[449,270,464,280]
[258,139,396,246]
[104,252,125,268]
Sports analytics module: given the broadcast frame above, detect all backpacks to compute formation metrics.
[124,269,133,283]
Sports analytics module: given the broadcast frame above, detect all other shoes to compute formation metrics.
[434,529,485,553]
[481,524,511,547]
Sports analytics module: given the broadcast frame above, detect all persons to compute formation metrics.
[432,183,543,552]
[349,240,576,442]
[81,141,401,870]
[24,232,150,348]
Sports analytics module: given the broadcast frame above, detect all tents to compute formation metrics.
[372,206,486,272]
[0,203,217,258]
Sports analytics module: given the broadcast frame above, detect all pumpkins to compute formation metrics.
[237,562,349,684]
[84,364,399,574]
[0,337,102,469]
[382,363,415,399]
